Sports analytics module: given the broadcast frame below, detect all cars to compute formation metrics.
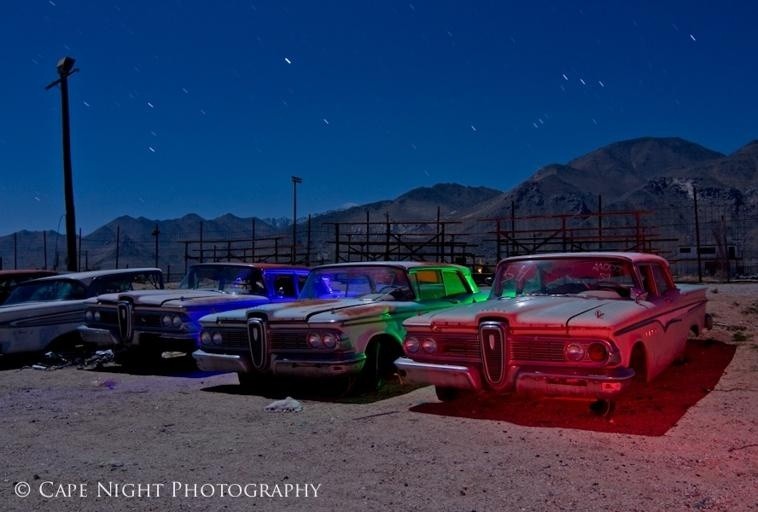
[74,258,365,354]
[0,266,166,359]
[189,256,529,382]
[392,243,712,405]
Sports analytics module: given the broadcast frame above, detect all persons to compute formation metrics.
[229,269,257,295]
